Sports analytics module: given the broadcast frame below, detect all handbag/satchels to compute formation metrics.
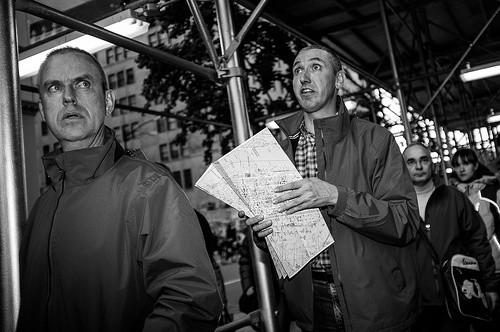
[439,253,492,324]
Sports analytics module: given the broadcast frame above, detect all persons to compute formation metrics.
[15,47,223,332]
[402,143,500,332]
[238,45,420,332]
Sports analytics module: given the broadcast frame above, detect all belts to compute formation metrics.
[312,270,334,282]
